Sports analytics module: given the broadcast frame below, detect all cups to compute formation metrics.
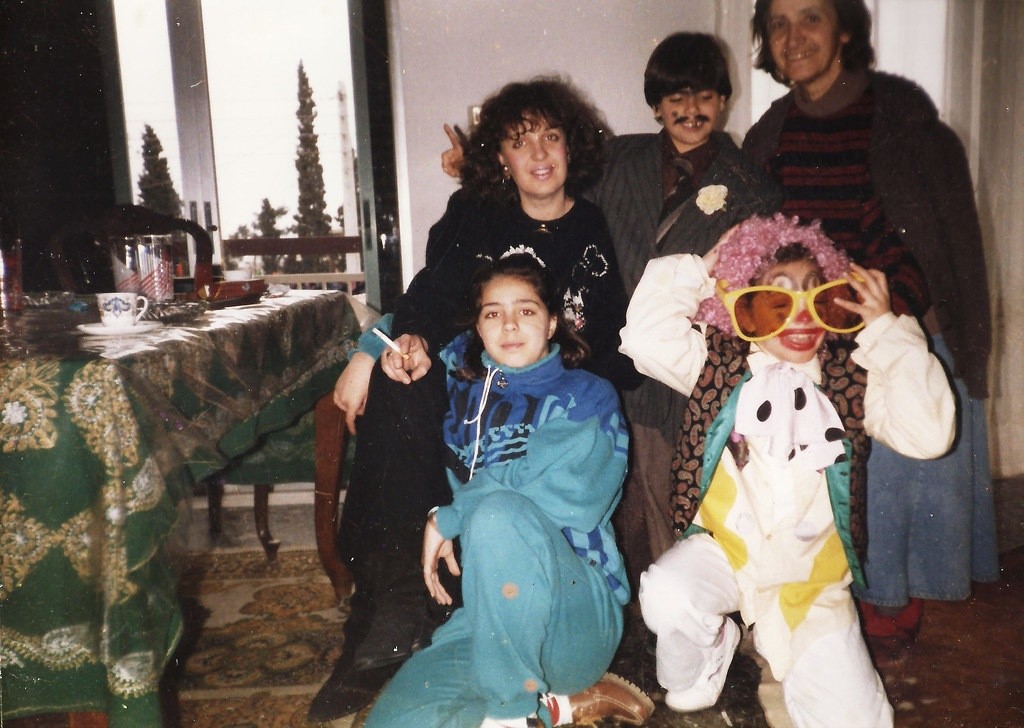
[109,237,139,292]
[222,272,252,281]
[0,239,23,310]
[95,293,148,327]
[139,235,173,301]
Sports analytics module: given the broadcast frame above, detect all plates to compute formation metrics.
[78,321,161,335]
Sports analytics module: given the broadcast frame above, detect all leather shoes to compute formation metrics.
[568,671,656,727]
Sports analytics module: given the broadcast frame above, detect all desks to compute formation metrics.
[0,288,362,728]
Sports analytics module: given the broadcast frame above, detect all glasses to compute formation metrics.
[715,272,867,342]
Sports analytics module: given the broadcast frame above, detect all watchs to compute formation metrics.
[428,506,441,532]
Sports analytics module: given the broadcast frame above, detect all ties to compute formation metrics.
[659,155,695,226]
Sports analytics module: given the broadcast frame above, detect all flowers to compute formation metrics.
[697,184,729,215]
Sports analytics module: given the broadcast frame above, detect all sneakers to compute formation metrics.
[665,614,740,712]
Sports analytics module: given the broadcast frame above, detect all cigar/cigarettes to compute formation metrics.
[372,327,410,360]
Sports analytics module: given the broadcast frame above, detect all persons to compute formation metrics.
[619,214,956,728]
[442,31,780,680]
[334,244,654,727]
[738,0,1001,657]
[307,74,644,720]
